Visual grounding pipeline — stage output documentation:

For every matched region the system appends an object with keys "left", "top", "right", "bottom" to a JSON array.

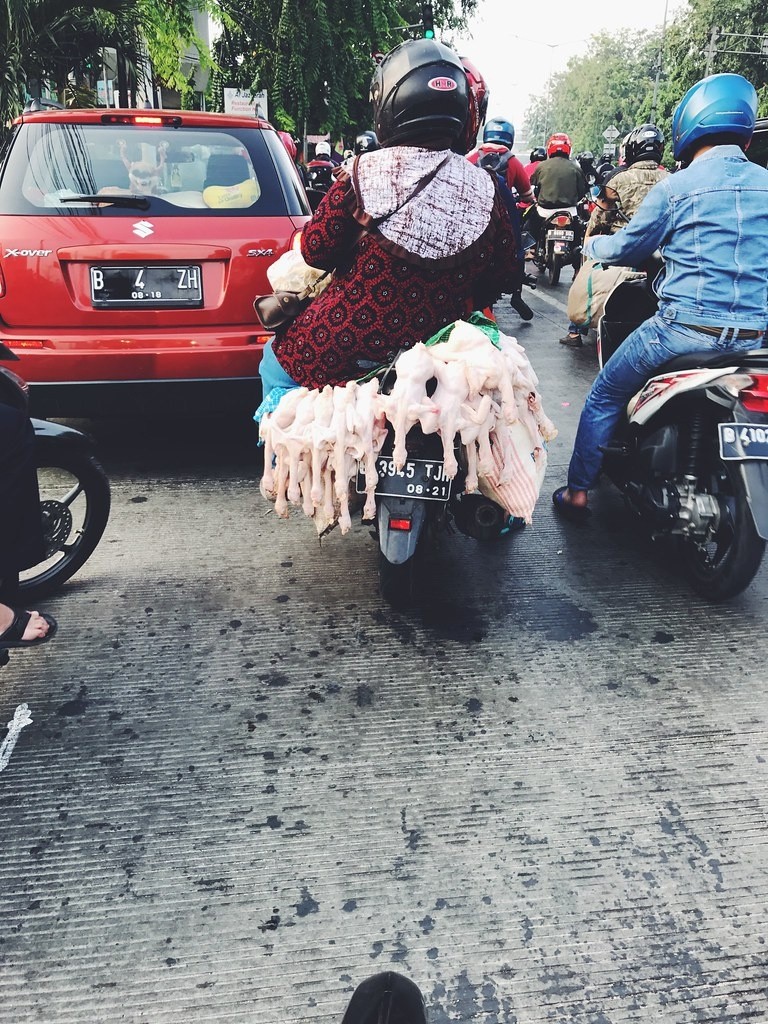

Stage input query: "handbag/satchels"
[{"left": 252, "top": 290, "right": 316, "bottom": 332}]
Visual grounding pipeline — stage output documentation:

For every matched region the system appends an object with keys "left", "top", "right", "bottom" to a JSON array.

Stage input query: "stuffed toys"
[
  {"left": 203, "top": 179, "right": 261, "bottom": 208},
  {"left": 120, "top": 146, "right": 164, "bottom": 194}
]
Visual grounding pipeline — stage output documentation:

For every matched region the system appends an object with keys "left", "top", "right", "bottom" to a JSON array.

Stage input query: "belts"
[{"left": 683, "top": 322, "right": 762, "bottom": 341}]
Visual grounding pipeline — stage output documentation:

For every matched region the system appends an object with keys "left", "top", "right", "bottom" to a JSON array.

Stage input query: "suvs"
[{"left": 0, "top": 102, "right": 327, "bottom": 420}]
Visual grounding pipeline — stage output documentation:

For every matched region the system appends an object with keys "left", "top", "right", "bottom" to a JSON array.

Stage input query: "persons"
[
  {"left": 259, "top": 39, "right": 673, "bottom": 418},
  {"left": 551, "top": 72, "right": 767, "bottom": 519},
  {"left": 0, "top": 403, "right": 58, "bottom": 650}
]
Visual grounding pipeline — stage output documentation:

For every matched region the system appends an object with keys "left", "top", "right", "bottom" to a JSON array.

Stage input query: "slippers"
[
  {"left": 553, "top": 486, "right": 591, "bottom": 519},
  {"left": 0, "top": 609, "right": 57, "bottom": 648}
]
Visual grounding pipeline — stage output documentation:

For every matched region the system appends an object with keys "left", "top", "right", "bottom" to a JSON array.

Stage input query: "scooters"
[
  {"left": 590, "top": 182, "right": 767, "bottom": 604},
  {"left": 490, "top": 187, "right": 599, "bottom": 312}
]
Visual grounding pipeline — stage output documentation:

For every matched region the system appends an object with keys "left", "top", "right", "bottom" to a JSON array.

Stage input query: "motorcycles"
[
  {"left": 351, "top": 410, "right": 510, "bottom": 610},
  {"left": 0, "top": 340, "right": 114, "bottom": 672}
]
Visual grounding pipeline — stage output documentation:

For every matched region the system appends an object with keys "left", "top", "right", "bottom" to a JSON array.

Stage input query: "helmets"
[
  {"left": 622, "top": 124, "right": 665, "bottom": 168},
  {"left": 530, "top": 147, "right": 547, "bottom": 164},
  {"left": 316, "top": 142, "right": 331, "bottom": 157},
  {"left": 369, "top": 39, "right": 469, "bottom": 151},
  {"left": 354, "top": 130, "right": 382, "bottom": 156},
  {"left": 575, "top": 151, "right": 593, "bottom": 170},
  {"left": 279, "top": 132, "right": 297, "bottom": 159},
  {"left": 602, "top": 154, "right": 611, "bottom": 164},
  {"left": 482, "top": 118, "right": 513, "bottom": 150},
  {"left": 672, "top": 73, "right": 757, "bottom": 161},
  {"left": 343, "top": 150, "right": 354, "bottom": 160},
  {"left": 547, "top": 132, "right": 570, "bottom": 160},
  {"left": 461, "top": 58, "right": 487, "bottom": 155}
]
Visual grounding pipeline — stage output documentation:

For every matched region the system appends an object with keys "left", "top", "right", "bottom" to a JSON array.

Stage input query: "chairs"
[
  {"left": 90, "top": 159, "right": 130, "bottom": 189},
  {"left": 206, "top": 154, "right": 250, "bottom": 187}
]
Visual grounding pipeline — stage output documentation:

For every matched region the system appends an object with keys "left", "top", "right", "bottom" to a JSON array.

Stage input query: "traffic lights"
[{"left": 422, "top": 4, "right": 435, "bottom": 39}]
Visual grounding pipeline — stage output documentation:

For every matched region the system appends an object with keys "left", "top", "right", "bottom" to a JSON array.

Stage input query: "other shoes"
[
  {"left": 511, "top": 296, "right": 534, "bottom": 320},
  {"left": 559, "top": 333, "right": 582, "bottom": 347}
]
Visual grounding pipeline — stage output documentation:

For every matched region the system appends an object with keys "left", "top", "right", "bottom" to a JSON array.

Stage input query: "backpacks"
[{"left": 475, "top": 149, "right": 515, "bottom": 187}]
